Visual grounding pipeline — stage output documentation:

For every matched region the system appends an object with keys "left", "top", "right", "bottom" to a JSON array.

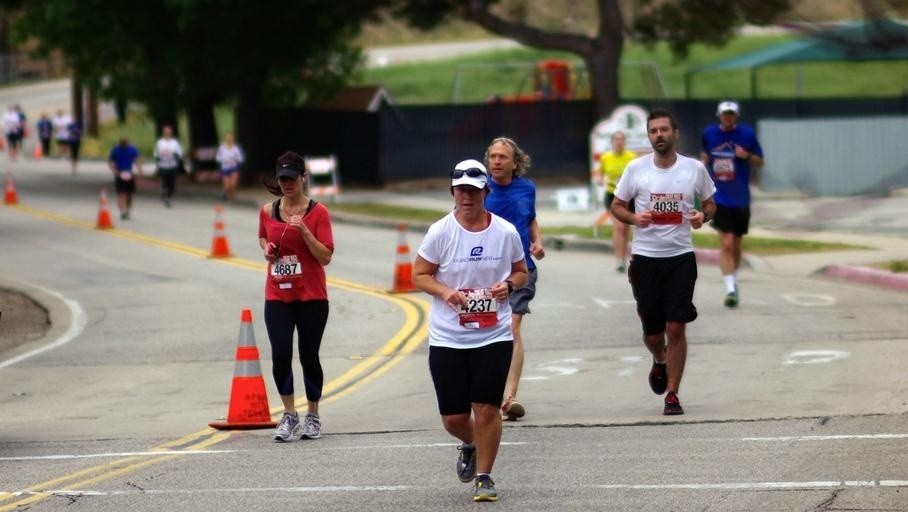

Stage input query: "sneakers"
[
  {"left": 471, "top": 473, "right": 499, "bottom": 503},
  {"left": 299, "top": 413, "right": 323, "bottom": 441},
  {"left": 272, "top": 411, "right": 301, "bottom": 443},
  {"left": 648, "top": 345, "right": 669, "bottom": 396},
  {"left": 663, "top": 391, "right": 684, "bottom": 415},
  {"left": 500, "top": 395, "right": 526, "bottom": 422},
  {"left": 723, "top": 284, "right": 740, "bottom": 307},
  {"left": 455, "top": 442, "right": 478, "bottom": 484}
]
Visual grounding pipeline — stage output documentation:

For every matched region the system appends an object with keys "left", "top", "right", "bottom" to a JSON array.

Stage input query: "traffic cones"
[
  {"left": 206, "top": 309, "right": 281, "bottom": 429},
  {"left": 94, "top": 184, "right": 117, "bottom": 230},
  {"left": 0, "top": 135, "right": 5, "bottom": 151},
  {"left": 23, "top": 122, "right": 29, "bottom": 139},
  {"left": 207, "top": 204, "right": 234, "bottom": 259},
  {"left": 384, "top": 223, "right": 425, "bottom": 294},
  {"left": 33, "top": 139, "right": 44, "bottom": 159},
  {"left": 3, "top": 170, "right": 22, "bottom": 205}
]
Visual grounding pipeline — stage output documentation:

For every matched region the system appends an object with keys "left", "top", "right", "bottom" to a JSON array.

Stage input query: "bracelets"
[
  {"left": 744, "top": 153, "right": 752, "bottom": 163},
  {"left": 505, "top": 279, "right": 514, "bottom": 295},
  {"left": 703, "top": 211, "right": 710, "bottom": 223}
]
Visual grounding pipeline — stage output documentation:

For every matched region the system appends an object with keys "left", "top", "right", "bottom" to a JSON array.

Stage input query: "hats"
[
  {"left": 451, "top": 158, "right": 488, "bottom": 189},
  {"left": 716, "top": 100, "right": 739, "bottom": 115},
  {"left": 275, "top": 151, "right": 306, "bottom": 181}
]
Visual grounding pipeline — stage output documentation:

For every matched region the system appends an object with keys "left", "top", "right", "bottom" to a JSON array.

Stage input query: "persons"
[
  {"left": 149, "top": 124, "right": 184, "bottom": 206},
  {"left": 107, "top": 136, "right": 142, "bottom": 220},
  {"left": 212, "top": 130, "right": 246, "bottom": 202},
  {"left": 1, "top": 101, "right": 84, "bottom": 178},
  {"left": 411, "top": 158, "right": 529, "bottom": 502},
  {"left": 594, "top": 132, "right": 637, "bottom": 273},
  {"left": 699, "top": 97, "right": 767, "bottom": 308},
  {"left": 258, "top": 151, "right": 335, "bottom": 442},
  {"left": 482, "top": 137, "right": 546, "bottom": 421},
  {"left": 609, "top": 111, "right": 718, "bottom": 414}
]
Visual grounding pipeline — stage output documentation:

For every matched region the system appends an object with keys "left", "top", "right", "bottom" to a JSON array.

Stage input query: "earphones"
[{"left": 302, "top": 177, "right": 304, "bottom": 182}]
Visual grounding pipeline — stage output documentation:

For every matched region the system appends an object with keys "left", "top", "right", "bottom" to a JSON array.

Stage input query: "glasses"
[{"left": 449, "top": 167, "right": 487, "bottom": 180}]
